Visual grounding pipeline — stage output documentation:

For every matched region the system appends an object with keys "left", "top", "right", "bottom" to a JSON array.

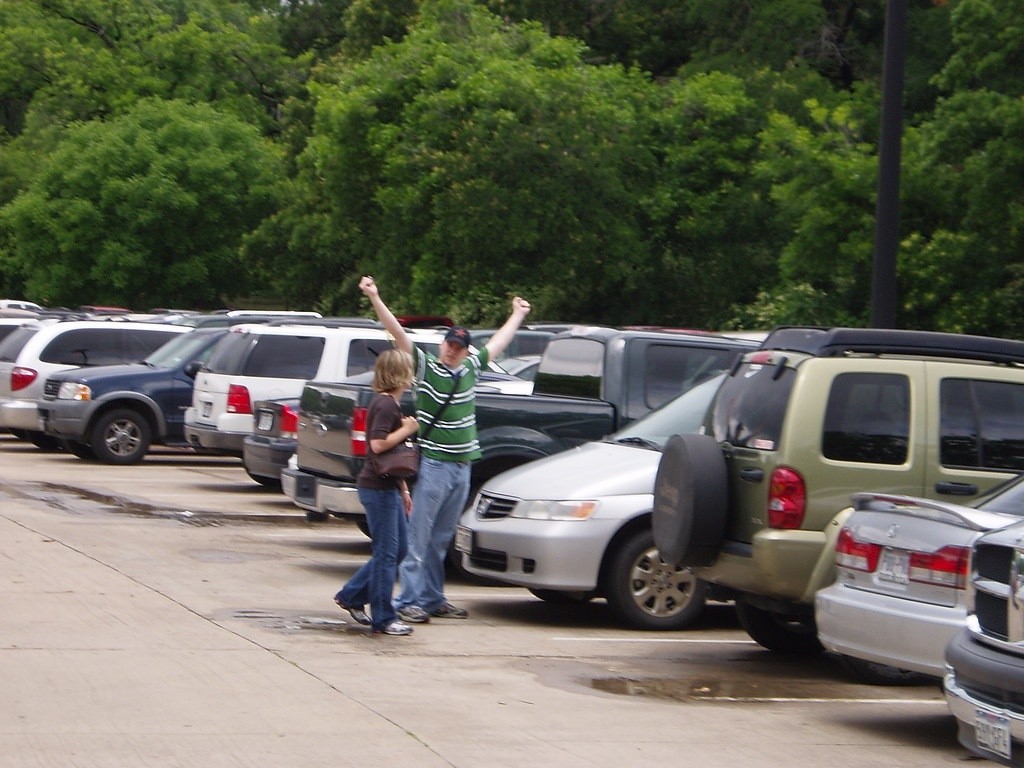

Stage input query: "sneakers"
[
  {"left": 334, "top": 594, "right": 372, "bottom": 625},
  {"left": 395, "top": 605, "right": 430, "bottom": 624},
  {"left": 372, "top": 619, "right": 413, "bottom": 636},
  {"left": 430, "top": 603, "right": 468, "bottom": 618}
]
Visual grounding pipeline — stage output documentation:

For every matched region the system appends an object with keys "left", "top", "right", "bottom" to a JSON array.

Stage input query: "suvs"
[
  {"left": 178, "top": 320, "right": 514, "bottom": 468},
  {"left": 652, "top": 328, "right": 1024, "bottom": 667}
]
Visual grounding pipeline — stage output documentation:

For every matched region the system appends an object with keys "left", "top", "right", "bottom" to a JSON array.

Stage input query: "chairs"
[{"left": 858, "top": 410, "right": 894, "bottom": 434}]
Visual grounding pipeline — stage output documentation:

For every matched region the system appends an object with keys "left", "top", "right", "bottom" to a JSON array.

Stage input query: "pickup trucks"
[{"left": 277, "top": 324, "right": 766, "bottom": 588}]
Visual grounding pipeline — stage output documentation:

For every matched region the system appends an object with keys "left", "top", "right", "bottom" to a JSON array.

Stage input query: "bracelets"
[{"left": 401, "top": 491, "right": 410, "bottom": 495}]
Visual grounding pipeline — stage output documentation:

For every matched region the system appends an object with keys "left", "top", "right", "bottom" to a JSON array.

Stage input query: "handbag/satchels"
[{"left": 371, "top": 397, "right": 419, "bottom": 479}]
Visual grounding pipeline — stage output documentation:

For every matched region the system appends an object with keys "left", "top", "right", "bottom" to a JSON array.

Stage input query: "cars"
[
  {"left": 461, "top": 326, "right": 555, "bottom": 382},
  {"left": 454, "top": 368, "right": 731, "bottom": 633},
  {"left": 812, "top": 470, "right": 1024, "bottom": 684},
  {"left": 243, "top": 372, "right": 369, "bottom": 485},
  {"left": 0, "top": 297, "right": 322, "bottom": 467},
  {"left": 941, "top": 517, "right": 1024, "bottom": 768}
]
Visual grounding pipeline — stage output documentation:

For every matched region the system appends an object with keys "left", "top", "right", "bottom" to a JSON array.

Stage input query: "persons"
[
  {"left": 359, "top": 276, "right": 531, "bottom": 623},
  {"left": 333, "top": 351, "right": 420, "bottom": 636}
]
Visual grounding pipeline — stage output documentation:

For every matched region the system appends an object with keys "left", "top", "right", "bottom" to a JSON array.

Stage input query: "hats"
[{"left": 445, "top": 326, "right": 471, "bottom": 347}]
